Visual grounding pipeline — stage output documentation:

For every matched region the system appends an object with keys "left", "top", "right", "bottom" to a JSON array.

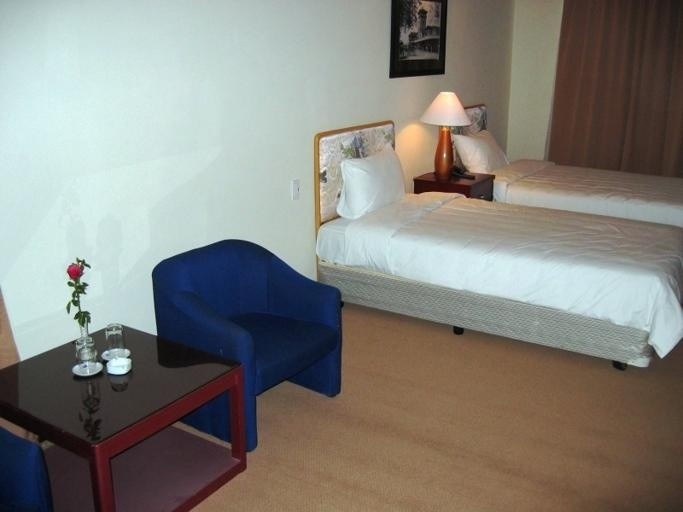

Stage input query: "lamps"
[{"left": 417, "top": 91, "right": 471, "bottom": 180}]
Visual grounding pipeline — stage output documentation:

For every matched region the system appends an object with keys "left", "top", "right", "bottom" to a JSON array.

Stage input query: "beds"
[
  {"left": 446, "top": 103, "right": 681, "bottom": 227},
  {"left": 312, "top": 120, "right": 682, "bottom": 371}
]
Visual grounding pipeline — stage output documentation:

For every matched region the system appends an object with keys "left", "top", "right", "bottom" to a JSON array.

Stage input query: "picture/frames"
[{"left": 388, "top": 0, "right": 448, "bottom": 78}]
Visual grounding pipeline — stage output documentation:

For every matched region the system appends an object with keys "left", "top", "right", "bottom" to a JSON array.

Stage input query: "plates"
[{"left": 72, "top": 348, "right": 131, "bottom": 378}]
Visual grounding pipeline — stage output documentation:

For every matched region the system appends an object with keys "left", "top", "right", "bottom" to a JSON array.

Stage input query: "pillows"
[
  {"left": 335, "top": 142, "right": 406, "bottom": 218},
  {"left": 453, "top": 128, "right": 510, "bottom": 174}
]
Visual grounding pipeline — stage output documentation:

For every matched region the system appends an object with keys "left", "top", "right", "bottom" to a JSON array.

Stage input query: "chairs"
[
  {"left": 0, "top": 424, "right": 55, "bottom": 511},
  {"left": 151, "top": 240, "right": 343, "bottom": 450}
]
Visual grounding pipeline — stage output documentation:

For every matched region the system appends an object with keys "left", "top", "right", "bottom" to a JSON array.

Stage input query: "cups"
[{"left": 74, "top": 324, "right": 126, "bottom": 374}]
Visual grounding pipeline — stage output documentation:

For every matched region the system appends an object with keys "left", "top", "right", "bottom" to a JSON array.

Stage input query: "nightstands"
[{"left": 414, "top": 171, "right": 496, "bottom": 202}]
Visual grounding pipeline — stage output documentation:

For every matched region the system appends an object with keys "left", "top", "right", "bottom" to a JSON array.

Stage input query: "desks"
[{"left": 0, "top": 322, "right": 246, "bottom": 512}]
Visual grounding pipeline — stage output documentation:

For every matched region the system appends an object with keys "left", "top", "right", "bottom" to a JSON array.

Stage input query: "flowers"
[{"left": 65, "top": 257, "right": 93, "bottom": 325}]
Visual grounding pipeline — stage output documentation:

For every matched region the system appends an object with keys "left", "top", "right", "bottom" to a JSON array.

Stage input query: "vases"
[{"left": 74, "top": 319, "right": 98, "bottom": 372}]
